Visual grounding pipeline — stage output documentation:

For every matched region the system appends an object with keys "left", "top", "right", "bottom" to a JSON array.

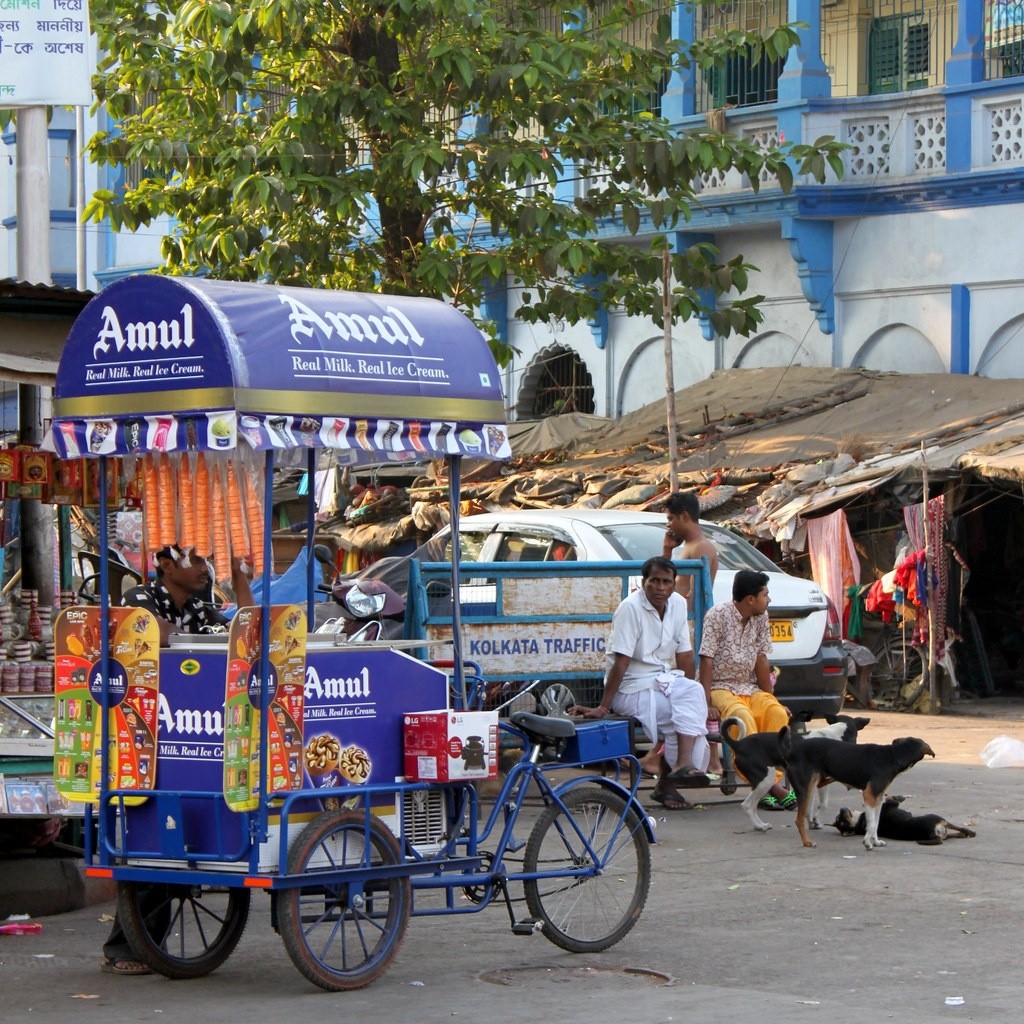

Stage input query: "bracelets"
[{"left": 598, "top": 705, "right": 610, "bottom": 716}]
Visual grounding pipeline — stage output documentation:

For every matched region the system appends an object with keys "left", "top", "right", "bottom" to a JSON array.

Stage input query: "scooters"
[{"left": 79, "top": 539, "right": 451, "bottom": 641}]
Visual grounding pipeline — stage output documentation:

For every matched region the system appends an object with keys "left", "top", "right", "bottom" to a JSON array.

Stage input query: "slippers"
[
  {"left": 648, "top": 788, "right": 695, "bottom": 810},
  {"left": 667, "top": 764, "right": 711, "bottom": 787},
  {"left": 780, "top": 789, "right": 798, "bottom": 810},
  {"left": 757, "top": 796, "right": 785, "bottom": 811},
  {"left": 101, "top": 956, "right": 152, "bottom": 974},
  {"left": 620, "top": 757, "right": 662, "bottom": 779}
]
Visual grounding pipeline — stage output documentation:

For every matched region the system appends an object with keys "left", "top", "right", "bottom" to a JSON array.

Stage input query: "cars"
[{"left": 413, "top": 509, "right": 849, "bottom": 717}]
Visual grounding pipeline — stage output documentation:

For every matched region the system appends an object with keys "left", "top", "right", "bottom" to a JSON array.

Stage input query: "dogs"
[{"left": 719, "top": 715, "right": 977, "bottom": 851}]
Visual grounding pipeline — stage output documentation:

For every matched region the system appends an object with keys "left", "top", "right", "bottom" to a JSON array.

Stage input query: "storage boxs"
[
  {"left": 564, "top": 720, "right": 631, "bottom": 765},
  {"left": 402, "top": 708, "right": 499, "bottom": 784}
]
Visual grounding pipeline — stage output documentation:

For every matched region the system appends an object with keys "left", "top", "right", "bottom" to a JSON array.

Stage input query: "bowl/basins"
[{"left": 0, "top": 587, "right": 79, "bottom": 694}]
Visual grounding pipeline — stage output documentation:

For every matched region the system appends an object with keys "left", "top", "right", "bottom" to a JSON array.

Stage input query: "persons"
[
  {"left": 696, "top": 568, "right": 796, "bottom": 810},
  {"left": 661, "top": 492, "right": 718, "bottom": 645},
  {"left": 565, "top": 557, "right": 712, "bottom": 808},
  {"left": 99, "top": 540, "right": 257, "bottom": 975}
]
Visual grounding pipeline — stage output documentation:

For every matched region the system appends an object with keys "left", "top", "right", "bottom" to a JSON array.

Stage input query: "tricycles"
[{"left": 40, "top": 273, "right": 658, "bottom": 992}]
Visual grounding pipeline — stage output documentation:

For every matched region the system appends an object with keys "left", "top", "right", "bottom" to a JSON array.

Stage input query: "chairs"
[{"left": 79, "top": 549, "right": 142, "bottom": 608}]
